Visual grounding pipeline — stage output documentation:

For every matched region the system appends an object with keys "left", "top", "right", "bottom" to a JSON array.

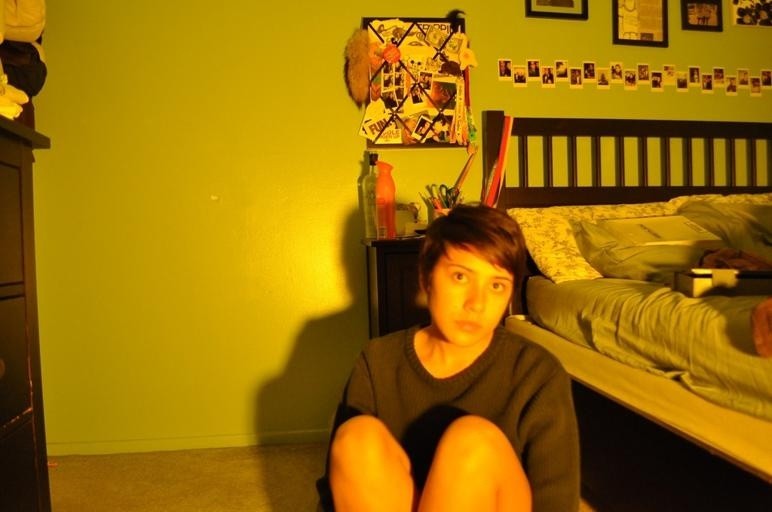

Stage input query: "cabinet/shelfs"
[{"left": 0, "top": 116, "right": 52, "bottom": 509}]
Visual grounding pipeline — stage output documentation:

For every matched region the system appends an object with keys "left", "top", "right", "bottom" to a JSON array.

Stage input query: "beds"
[{"left": 480, "top": 108, "right": 771, "bottom": 512}]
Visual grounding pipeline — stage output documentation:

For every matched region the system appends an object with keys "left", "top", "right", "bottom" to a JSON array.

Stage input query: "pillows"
[{"left": 505, "top": 191, "right": 771, "bottom": 287}]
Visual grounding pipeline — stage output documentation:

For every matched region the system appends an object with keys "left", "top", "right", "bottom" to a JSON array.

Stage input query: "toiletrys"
[
  {"left": 361, "top": 151, "right": 378, "bottom": 237},
  {"left": 373, "top": 160, "right": 397, "bottom": 238}
]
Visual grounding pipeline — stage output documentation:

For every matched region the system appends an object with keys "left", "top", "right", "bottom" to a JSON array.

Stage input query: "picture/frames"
[{"left": 523, "top": 0, "right": 723, "bottom": 48}]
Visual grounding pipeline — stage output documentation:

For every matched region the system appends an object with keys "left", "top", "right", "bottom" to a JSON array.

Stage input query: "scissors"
[{"left": 440, "top": 184, "right": 459, "bottom": 208}]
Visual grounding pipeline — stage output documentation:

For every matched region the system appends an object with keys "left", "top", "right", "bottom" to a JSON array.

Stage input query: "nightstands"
[{"left": 360, "top": 235, "right": 431, "bottom": 340}]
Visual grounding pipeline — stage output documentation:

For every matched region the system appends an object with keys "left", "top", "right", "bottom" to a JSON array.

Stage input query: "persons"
[
  {"left": 696, "top": 5, "right": 712, "bottom": 25},
  {"left": 314, "top": 202, "right": 582, "bottom": 512},
  {"left": 375, "top": 25, "right": 456, "bottom": 143},
  {"left": 499, "top": 61, "right": 771, "bottom": 93}
]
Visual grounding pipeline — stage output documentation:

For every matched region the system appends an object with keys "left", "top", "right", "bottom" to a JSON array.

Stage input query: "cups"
[{"left": 433, "top": 208, "right": 451, "bottom": 221}]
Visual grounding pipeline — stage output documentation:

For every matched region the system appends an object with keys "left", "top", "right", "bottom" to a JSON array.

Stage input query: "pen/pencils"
[{"left": 429, "top": 184, "right": 452, "bottom": 208}]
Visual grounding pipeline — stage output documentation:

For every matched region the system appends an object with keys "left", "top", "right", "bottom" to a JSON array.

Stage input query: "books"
[
  {"left": 673, "top": 268, "right": 772, "bottom": 297},
  {"left": 596, "top": 214, "right": 725, "bottom": 248}
]
